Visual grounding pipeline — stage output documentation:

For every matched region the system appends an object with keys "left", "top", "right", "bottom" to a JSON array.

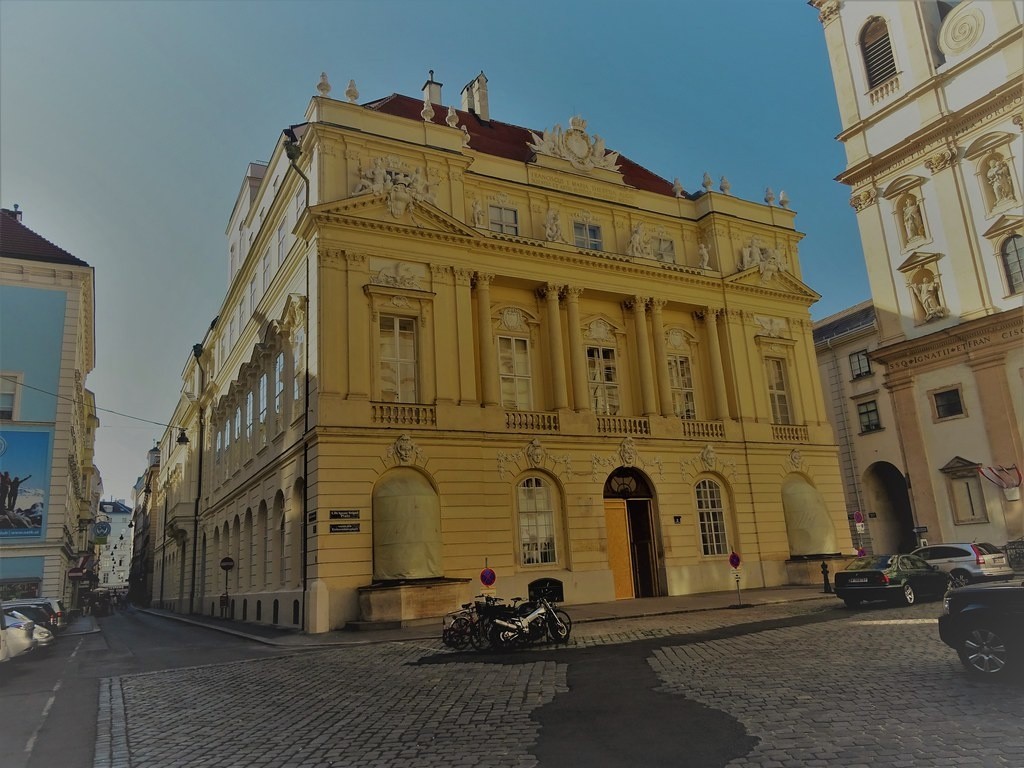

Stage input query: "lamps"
[
  {"left": 176, "top": 432, "right": 190, "bottom": 445},
  {"left": 127, "top": 521, "right": 134, "bottom": 528},
  {"left": 143, "top": 484, "right": 152, "bottom": 494}
]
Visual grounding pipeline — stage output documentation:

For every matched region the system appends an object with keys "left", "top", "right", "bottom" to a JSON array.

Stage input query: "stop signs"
[{"left": 220, "top": 557, "right": 234, "bottom": 571}]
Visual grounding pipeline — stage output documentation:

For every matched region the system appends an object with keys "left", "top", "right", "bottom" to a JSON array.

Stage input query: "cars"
[
  {"left": 0, "top": 596, "right": 72, "bottom": 663},
  {"left": 833, "top": 553, "right": 950, "bottom": 611},
  {"left": 937, "top": 583, "right": 1024, "bottom": 677}
]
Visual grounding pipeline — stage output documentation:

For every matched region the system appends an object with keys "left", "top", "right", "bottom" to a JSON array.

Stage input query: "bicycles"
[{"left": 440, "top": 582, "right": 572, "bottom": 655}]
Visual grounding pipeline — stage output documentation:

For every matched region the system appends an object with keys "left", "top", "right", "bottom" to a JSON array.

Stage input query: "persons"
[
  {"left": 698, "top": 243, "right": 713, "bottom": 270},
  {"left": 471, "top": 200, "right": 483, "bottom": 228},
  {"left": 408, "top": 167, "right": 438, "bottom": 207},
  {"left": 544, "top": 209, "right": 562, "bottom": 242},
  {"left": 901, "top": 196, "right": 925, "bottom": 241},
  {"left": 911, "top": 277, "right": 944, "bottom": 324},
  {"left": 351, "top": 156, "right": 393, "bottom": 198},
  {"left": 630, "top": 228, "right": 645, "bottom": 258},
  {"left": 987, "top": 159, "right": 1014, "bottom": 205},
  {"left": 82, "top": 588, "right": 128, "bottom": 618},
  {"left": 0, "top": 471, "right": 32, "bottom": 511},
  {"left": 749, "top": 234, "right": 765, "bottom": 266}
]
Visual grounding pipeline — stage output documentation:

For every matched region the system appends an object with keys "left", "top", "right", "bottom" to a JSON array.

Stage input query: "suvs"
[{"left": 907, "top": 537, "right": 1017, "bottom": 584}]
